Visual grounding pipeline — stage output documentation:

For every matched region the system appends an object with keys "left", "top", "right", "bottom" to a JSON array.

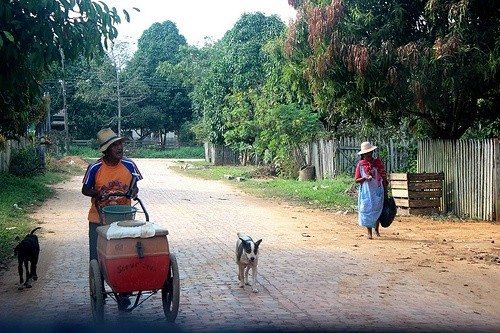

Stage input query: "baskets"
[{"left": 102, "top": 205, "right": 137, "bottom": 225}]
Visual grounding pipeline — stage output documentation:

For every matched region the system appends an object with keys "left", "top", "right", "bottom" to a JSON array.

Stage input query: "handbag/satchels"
[{"left": 379, "top": 197, "right": 397, "bottom": 227}]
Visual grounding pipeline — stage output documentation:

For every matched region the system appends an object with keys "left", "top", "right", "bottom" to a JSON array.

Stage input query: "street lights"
[{"left": 58, "top": 79, "right": 69, "bottom": 156}]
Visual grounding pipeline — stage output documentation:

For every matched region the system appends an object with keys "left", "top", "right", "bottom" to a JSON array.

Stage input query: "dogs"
[
  {"left": 235, "top": 232, "right": 263, "bottom": 293},
  {"left": 14, "top": 227, "right": 42, "bottom": 290}
]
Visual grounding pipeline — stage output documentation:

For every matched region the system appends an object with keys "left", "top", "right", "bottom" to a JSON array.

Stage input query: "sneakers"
[{"left": 117, "top": 297, "right": 131, "bottom": 310}]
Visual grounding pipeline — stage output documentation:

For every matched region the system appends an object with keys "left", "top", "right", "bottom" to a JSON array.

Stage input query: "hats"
[
  {"left": 358, "top": 142, "right": 378, "bottom": 155},
  {"left": 96, "top": 127, "right": 129, "bottom": 154}
]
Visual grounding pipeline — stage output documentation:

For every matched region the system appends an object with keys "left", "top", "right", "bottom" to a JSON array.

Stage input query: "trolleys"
[{"left": 88, "top": 192, "right": 180, "bottom": 323}]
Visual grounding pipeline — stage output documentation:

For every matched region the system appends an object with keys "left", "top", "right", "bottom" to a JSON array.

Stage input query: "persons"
[
  {"left": 81, "top": 127, "right": 143, "bottom": 311},
  {"left": 354, "top": 142, "right": 389, "bottom": 239}
]
